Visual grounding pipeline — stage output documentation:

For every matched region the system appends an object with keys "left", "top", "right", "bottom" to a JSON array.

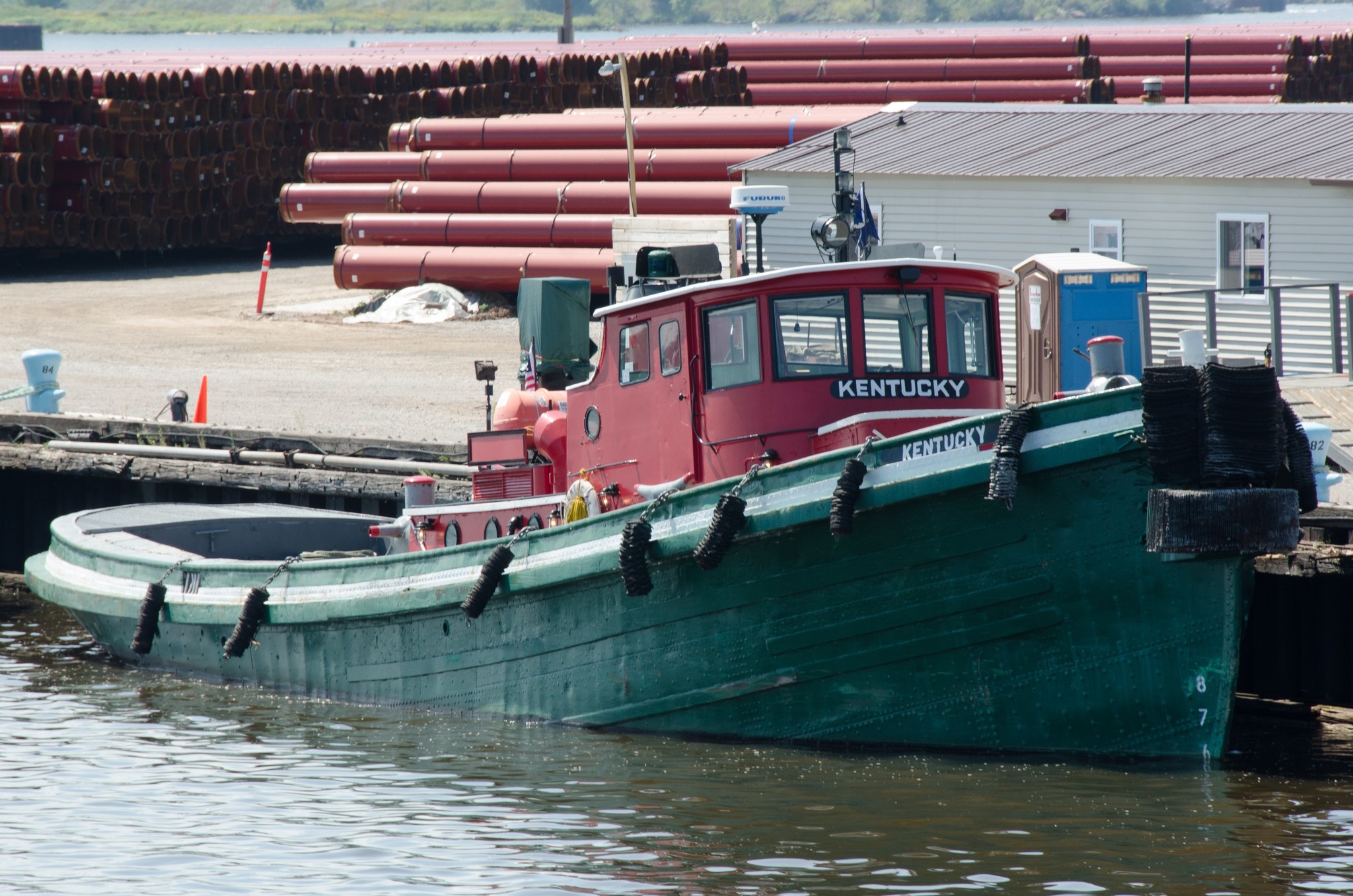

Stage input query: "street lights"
[
  {"left": 597, "top": 60, "right": 640, "bottom": 219},
  {"left": 832, "top": 127, "right": 853, "bottom": 263}
]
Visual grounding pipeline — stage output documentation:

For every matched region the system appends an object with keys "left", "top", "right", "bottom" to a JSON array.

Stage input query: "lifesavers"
[{"left": 561, "top": 478, "right": 610, "bottom": 524}]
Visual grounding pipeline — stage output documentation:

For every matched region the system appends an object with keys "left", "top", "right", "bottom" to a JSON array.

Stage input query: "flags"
[{"left": 525, "top": 342, "right": 539, "bottom": 391}]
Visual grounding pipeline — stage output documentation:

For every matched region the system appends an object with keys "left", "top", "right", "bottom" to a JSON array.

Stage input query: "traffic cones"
[{"left": 194, "top": 376, "right": 208, "bottom": 424}]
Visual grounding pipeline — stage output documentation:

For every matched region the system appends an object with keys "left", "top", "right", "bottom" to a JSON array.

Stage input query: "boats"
[{"left": 26, "top": 183, "right": 1257, "bottom": 766}]
